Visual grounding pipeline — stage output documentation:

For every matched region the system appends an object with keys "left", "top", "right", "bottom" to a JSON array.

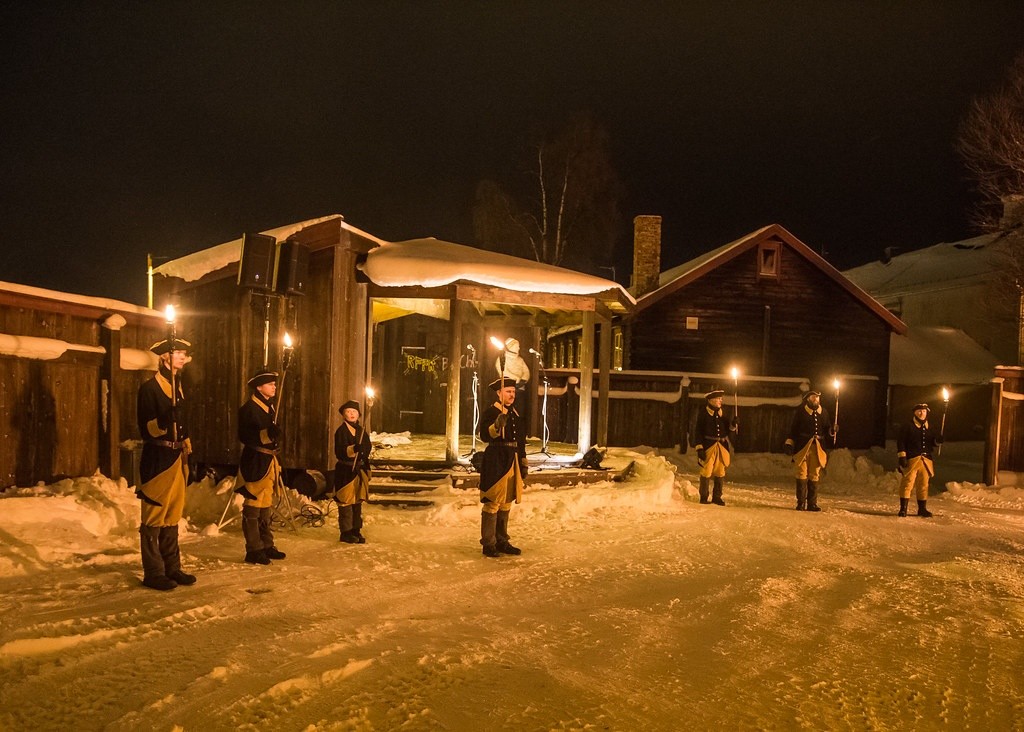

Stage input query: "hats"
[
  {"left": 339, "top": 400, "right": 362, "bottom": 417},
  {"left": 912, "top": 401, "right": 931, "bottom": 414},
  {"left": 248, "top": 371, "right": 279, "bottom": 387},
  {"left": 149, "top": 334, "right": 192, "bottom": 355},
  {"left": 802, "top": 389, "right": 821, "bottom": 402},
  {"left": 488, "top": 376, "right": 517, "bottom": 391},
  {"left": 703, "top": 389, "right": 725, "bottom": 400}
]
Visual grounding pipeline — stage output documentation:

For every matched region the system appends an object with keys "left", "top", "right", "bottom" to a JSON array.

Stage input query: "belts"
[
  {"left": 797, "top": 432, "right": 826, "bottom": 440},
  {"left": 338, "top": 458, "right": 365, "bottom": 466},
  {"left": 910, "top": 451, "right": 931, "bottom": 456},
  {"left": 489, "top": 440, "right": 518, "bottom": 448},
  {"left": 246, "top": 443, "right": 281, "bottom": 456},
  {"left": 143, "top": 437, "right": 183, "bottom": 450},
  {"left": 704, "top": 435, "right": 729, "bottom": 441}
]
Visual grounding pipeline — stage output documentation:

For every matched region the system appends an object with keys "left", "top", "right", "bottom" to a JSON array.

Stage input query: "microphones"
[
  {"left": 529, "top": 348, "right": 541, "bottom": 357},
  {"left": 467, "top": 344, "right": 475, "bottom": 352}
]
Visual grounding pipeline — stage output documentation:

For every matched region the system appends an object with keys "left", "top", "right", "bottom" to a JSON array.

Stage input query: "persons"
[
  {"left": 137, "top": 336, "right": 197, "bottom": 589},
  {"left": 786, "top": 388, "right": 839, "bottom": 512},
  {"left": 479, "top": 377, "right": 528, "bottom": 557},
  {"left": 334, "top": 400, "right": 373, "bottom": 543},
  {"left": 694, "top": 389, "right": 740, "bottom": 507},
  {"left": 896, "top": 402, "right": 944, "bottom": 517},
  {"left": 236, "top": 371, "right": 286, "bottom": 565},
  {"left": 496, "top": 338, "right": 530, "bottom": 446}
]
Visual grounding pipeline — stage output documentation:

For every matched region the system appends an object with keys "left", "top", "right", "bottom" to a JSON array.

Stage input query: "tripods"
[
  {"left": 462, "top": 352, "right": 479, "bottom": 460},
  {"left": 525, "top": 356, "right": 556, "bottom": 459}
]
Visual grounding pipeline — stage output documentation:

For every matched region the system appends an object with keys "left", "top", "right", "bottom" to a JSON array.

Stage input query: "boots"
[{"left": 139, "top": 475, "right": 937, "bottom": 591}]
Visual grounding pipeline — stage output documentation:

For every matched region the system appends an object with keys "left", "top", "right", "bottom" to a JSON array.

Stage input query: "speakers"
[{"left": 237, "top": 232, "right": 309, "bottom": 296}]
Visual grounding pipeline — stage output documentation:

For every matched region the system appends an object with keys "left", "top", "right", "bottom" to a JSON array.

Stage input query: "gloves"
[
  {"left": 267, "top": 424, "right": 281, "bottom": 440},
  {"left": 830, "top": 425, "right": 839, "bottom": 435},
  {"left": 697, "top": 448, "right": 706, "bottom": 461},
  {"left": 784, "top": 444, "right": 794, "bottom": 456},
  {"left": 731, "top": 416, "right": 741, "bottom": 428},
  {"left": 898, "top": 457, "right": 908, "bottom": 468},
  {"left": 520, "top": 465, "right": 528, "bottom": 479},
  {"left": 935, "top": 434, "right": 944, "bottom": 445},
  {"left": 354, "top": 444, "right": 364, "bottom": 453},
  {"left": 157, "top": 406, "right": 182, "bottom": 430},
  {"left": 495, "top": 413, "right": 510, "bottom": 428}
]
